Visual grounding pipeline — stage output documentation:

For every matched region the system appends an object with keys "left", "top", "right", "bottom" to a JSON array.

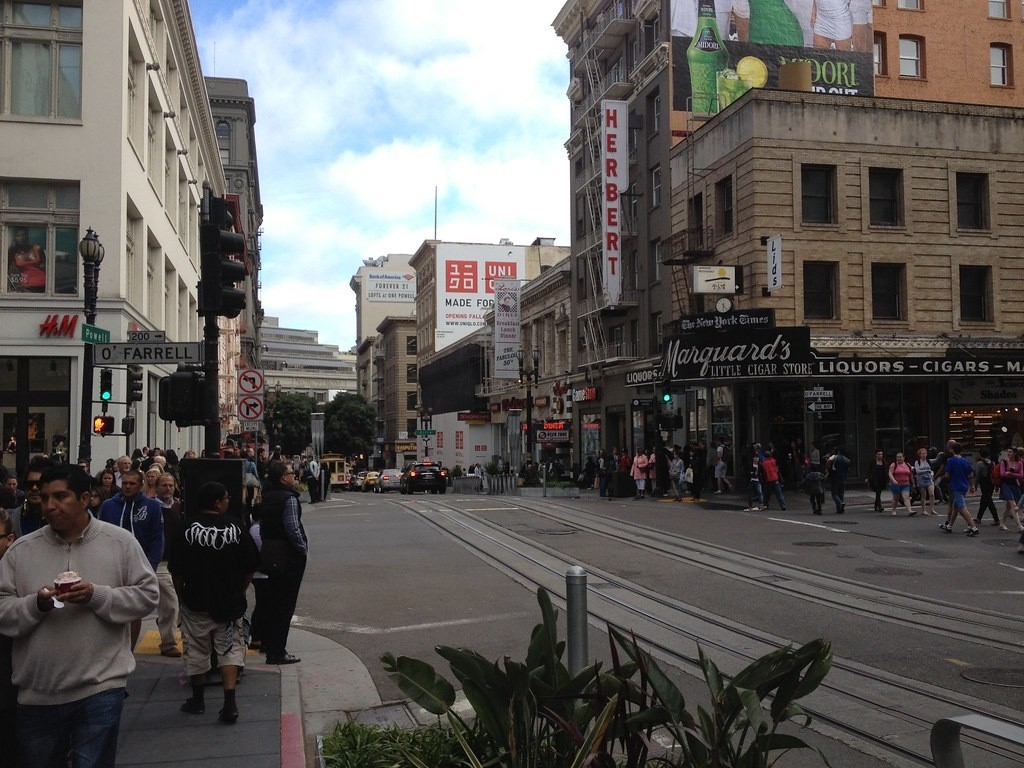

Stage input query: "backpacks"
[
  {"left": 978, "top": 459, "right": 995, "bottom": 480},
  {"left": 992, "top": 459, "right": 1007, "bottom": 485},
  {"left": 721, "top": 445, "right": 730, "bottom": 462}
]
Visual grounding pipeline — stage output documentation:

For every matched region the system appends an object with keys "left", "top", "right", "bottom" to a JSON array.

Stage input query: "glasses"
[
  {"left": 221, "top": 496, "right": 231, "bottom": 501},
  {"left": 286, "top": 471, "right": 295, "bottom": 476}
]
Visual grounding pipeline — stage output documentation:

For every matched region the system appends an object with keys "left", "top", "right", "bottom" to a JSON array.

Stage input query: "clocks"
[{"left": 715, "top": 298, "right": 733, "bottom": 312}]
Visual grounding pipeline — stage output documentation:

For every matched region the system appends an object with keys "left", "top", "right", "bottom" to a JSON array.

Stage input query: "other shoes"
[
  {"left": 749, "top": 501, "right": 938, "bottom": 517},
  {"left": 973, "top": 519, "right": 981, "bottom": 523},
  {"left": 181, "top": 698, "right": 204, "bottom": 714},
  {"left": 991, "top": 522, "right": 1000, "bottom": 526},
  {"left": 1018, "top": 543, "right": 1024, "bottom": 551},
  {"left": 219, "top": 707, "right": 239, "bottom": 721},
  {"left": 161, "top": 648, "right": 181, "bottom": 657},
  {"left": 714, "top": 490, "right": 722, "bottom": 493},
  {"left": 266, "top": 652, "right": 301, "bottom": 663},
  {"left": 249, "top": 641, "right": 261, "bottom": 649},
  {"left": 1000, "top": 525, "right": 1010, "bottom": 531},
  {"left": 1018, "top": 526, "right": 1024, "bottom": 532}
]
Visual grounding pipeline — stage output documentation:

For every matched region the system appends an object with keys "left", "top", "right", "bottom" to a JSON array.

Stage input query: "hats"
[
  {"left": 753, "top": 443, "right": 761, "bottom": 449},
  {"left": 765, "top": 451, "right": 771, "bottom": 455}
]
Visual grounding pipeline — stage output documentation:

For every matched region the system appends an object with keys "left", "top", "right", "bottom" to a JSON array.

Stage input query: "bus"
[{"left": 318, "top": 453, "right": 348, "bottom": 491}]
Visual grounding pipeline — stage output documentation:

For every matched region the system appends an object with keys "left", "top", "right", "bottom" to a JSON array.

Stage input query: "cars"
[
  {"left": 373, "top": 469, "right": 404, "bottom": 493},
  {"left": 346, "top": 465, "right": 380, "bottom": 493}
]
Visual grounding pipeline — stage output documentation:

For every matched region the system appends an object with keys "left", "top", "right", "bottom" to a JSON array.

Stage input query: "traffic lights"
[
  {"left": 201, "top": 223, "right": 247, "bottom": 309},
  {"left": 100, "top": 369, "right": 113, "bottom": 400},
  {"left": 660, "top": 375, "right": 673, "bottom": 403},
  {"left": 127, "top": 365, "right": 144, "bottom": 402},
  {"left": 94, "top": 415, "right": 109, "bottom": 432},
  {"left": 210, "top": 197, "right": 241, "bottom": 319}
]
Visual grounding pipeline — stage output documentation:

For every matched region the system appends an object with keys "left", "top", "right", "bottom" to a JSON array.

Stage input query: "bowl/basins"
[{"left": 53, "top": 577, "right": 82, "bottom": 596}]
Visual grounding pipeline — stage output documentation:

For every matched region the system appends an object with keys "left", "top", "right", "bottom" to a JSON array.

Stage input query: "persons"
[
  {"left": 939, "top": 437, "right": 1024, "bottom": 554},
  {"left": 7, "top": 419, "right": 38, "bottom": 450},
  {"left": 1, "top": 448, "right": 333, "bottom": 648},
  {"left": 670, "top": 0, "right": 873, "bottom": 53},
  {"left": 744, "top": 441, "right": 786, "bottom": 510},
  {"left": 55, "top": 441, "right": 68, "bottom": 463},
  {"left": 165, "top": 481, "right": 261, "bottom": 723},
  {"left": 9, "top": 227, "right": 69, "bottom": 293},
  {"left": 1, "top": 465, "right": 161, "bottom": 768},
  {"left": 865, "top": 447, "right": 943, "bottom": 515},
  {"left": 597, "top": 447, "right": 656, "bottom": 497},
  {"left": 259, "top": 464, "right": 308, "bottom": 666},
  {"left": 790, "top": 437, "right": 851, "bottom": 515},
  {"left": 463, "top": 463, "right": 484, "bottom": 489},
  {"left": 663, "top": 437, "right": 732, "bottom": 500}
]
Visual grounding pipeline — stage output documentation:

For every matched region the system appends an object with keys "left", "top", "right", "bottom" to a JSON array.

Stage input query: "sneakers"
[
  {"left": 967, "top": 529, "right": 979, "bottom": 537},
  {"left": 939, "top": 523, "right": 952, "bottom": 533},
  {"left": 963, "top": 527, "right": 971, "bottom": 533}
]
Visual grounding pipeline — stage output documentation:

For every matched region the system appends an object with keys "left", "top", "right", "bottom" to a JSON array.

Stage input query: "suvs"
[{"left": 399, "top": 461, "right": 447, "bottom": 495}]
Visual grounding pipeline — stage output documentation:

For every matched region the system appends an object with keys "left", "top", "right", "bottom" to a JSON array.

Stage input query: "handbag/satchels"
[
  {"left": 639, "top": 467, "right": 646, "bottom": 473},
  {"left": 685, "top": 468, "right": 693, "bottom": 483},
  {"left": 680, "top": 471, "right": 686, "bottom": 482},
  {"left": 671, "top": 473, "right": 679, "bottom": 481}
]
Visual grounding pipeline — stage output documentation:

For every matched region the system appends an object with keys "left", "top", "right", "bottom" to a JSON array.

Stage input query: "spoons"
[{"left": 43, "top": 587, "right": 64, "bottom": 609}]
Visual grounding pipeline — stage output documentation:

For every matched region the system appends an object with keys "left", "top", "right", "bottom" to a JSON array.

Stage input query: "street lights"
[
  {"left": 515, "top": 344, "right": 542, "bottom": 465},
  {"left": 78, "top": 227, "right": 107, "bottom": 474},
  {"left": 418, "top": 405, "right": 433, "bottom": 456}
]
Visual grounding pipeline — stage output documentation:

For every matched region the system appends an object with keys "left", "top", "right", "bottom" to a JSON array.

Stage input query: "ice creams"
[{"left": 53, "top": 571, "right": 82, "bottom": 583}]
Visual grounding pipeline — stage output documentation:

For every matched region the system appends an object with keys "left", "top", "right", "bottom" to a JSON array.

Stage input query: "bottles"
[{"left": 1010, "top": 464, "right": 1016, "bottom": 473}]
